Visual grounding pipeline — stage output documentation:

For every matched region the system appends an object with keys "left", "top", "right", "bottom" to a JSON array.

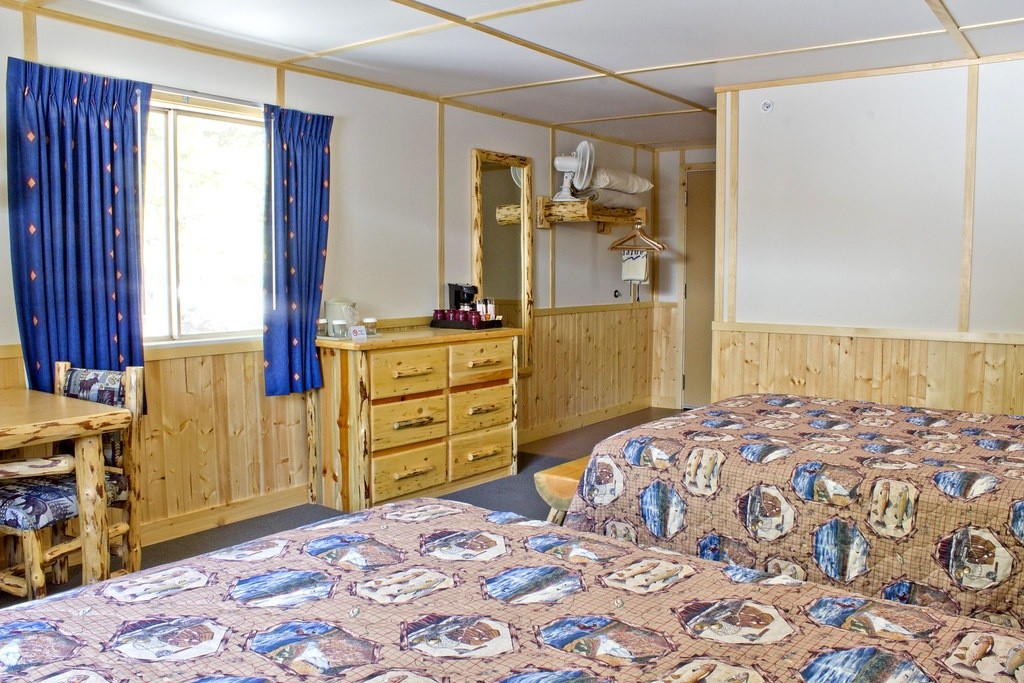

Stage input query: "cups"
[
  {"left": 433, "top": 309, "right": 445, "bottom": 320},
  {"left": 445, "top": 309, "right": 457, "bottom": 321},
  {"left": 331, "top": 320, "right": 348, "bottom": 337},
  {"left": 316, "top": 318, "right": 328, "bottom": 336},
  {"left": 468, "top": 311, "right": 481, "bottom": 327},
  {"left": 363, "top": 318, "right": 377, "bottom": 335},
  {"left": 455, "top": 310, "right": 468, "bottom": 321}
]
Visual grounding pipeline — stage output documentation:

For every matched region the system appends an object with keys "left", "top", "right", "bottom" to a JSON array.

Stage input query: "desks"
[{"left": 0, "top": 389, "right": 134, "bottom": 587}]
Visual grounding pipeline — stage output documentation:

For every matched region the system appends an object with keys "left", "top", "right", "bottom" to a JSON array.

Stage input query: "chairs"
[{"left": 0, "top": 360, "right": 147, "bottom": 605}]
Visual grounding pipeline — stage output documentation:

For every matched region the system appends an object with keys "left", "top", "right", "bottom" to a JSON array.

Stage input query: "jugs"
[
  {"left": 475, "top": 296, "right": 496, "bottom": 320},
  {"left": 324, "top": 295, "right": 356, "bottom": 337}
]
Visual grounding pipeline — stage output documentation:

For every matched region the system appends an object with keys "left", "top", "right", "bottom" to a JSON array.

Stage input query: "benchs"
[{"left": 532, "top": 451, "right": 590, "bottom": 526}]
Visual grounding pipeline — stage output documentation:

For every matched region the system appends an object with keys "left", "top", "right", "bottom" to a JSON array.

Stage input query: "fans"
[{"left": 552, "top": 140, "right": 595, "bottom": 201}]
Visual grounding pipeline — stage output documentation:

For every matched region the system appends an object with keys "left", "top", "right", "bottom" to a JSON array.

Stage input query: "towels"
[{"left": 620, "top": 250, "right": 650, "bottom": 285}]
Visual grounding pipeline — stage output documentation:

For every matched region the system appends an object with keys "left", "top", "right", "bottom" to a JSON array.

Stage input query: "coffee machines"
[{"left": 448, "top": 282, "right": 478, "bottom": 311}]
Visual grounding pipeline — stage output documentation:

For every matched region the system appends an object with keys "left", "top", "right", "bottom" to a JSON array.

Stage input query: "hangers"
[{"left": 606, "top": 218, "right": 667, "bottom": 254}]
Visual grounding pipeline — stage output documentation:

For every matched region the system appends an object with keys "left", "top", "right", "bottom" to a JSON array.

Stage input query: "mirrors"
[{"left": 470, "top": 148, "right": 537, "bottom": 379}]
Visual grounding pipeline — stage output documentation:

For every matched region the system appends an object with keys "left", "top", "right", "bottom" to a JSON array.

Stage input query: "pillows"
[{"left": 588, "top": 166, "right": 654, "bottom": 193}]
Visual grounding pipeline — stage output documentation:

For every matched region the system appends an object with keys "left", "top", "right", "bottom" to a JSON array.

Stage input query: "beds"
[
  {"left": 0, "top": 498, "right": 1023, "bottom": 683},
  {"left": 557, "top": 391, "right": 1024, "bottom": 632}
]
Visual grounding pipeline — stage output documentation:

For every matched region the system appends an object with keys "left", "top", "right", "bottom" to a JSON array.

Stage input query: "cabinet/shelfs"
[{"left": 305, "top": 323, "right": 525, "bottom": 516}]
